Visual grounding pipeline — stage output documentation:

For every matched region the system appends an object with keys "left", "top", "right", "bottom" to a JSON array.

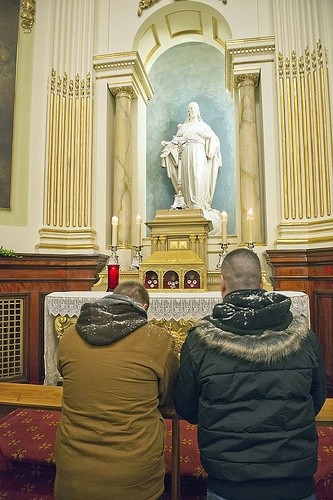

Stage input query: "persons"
[
  {"left": 159, "top": 100, "right": 222, "bottom": 211},
  {"left": 172, "top": 248, "right": 326, "bottom": 500},
  {"left": 52, "top": 281, "right": 179, "bottom": 500}
]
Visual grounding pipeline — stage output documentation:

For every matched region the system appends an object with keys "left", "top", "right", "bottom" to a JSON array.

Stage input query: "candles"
[
  {"left": 247, "top": 208, "right": 254, "bottom": 242},
  {"left": 135, "top": 214, "right": 141, "bottom": 244},
  {"left": 220, "top": 210, "right": 229, "bottom": 242},
  {"left": 111, "top": 216, "right": 119, "bottom": 246}
]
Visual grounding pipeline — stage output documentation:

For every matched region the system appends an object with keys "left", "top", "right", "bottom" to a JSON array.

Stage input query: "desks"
[{"left": 43, "top": 291, "right": 311, "bottom": 386}]
